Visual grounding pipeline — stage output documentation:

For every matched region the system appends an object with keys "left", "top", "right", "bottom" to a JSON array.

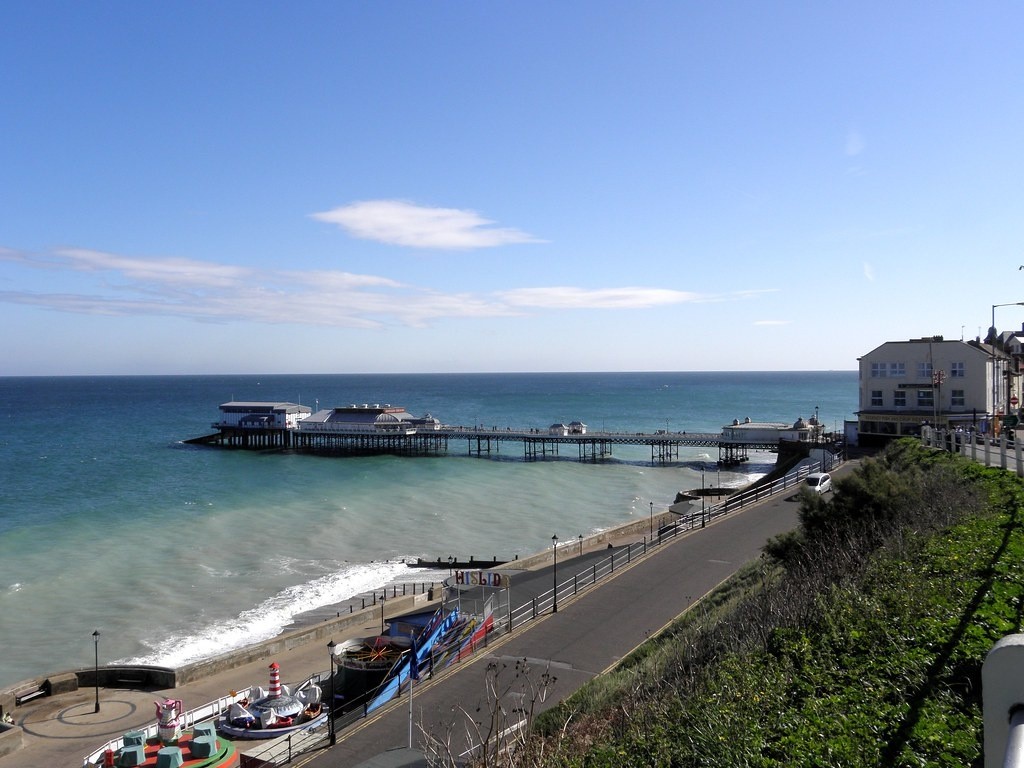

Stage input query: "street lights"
[
  {"left": 92, "top": 629, "right": 101, "bottom": 717},
  {"left": 579, "top": 534, "right": 583, "bottom": 557},
  {"left": 698, "top": 467, "right": 705, "bottom": 528},
  {"left": 821, "top": 423, "right": 827, "bottom": 473},
  {"left": 551, "top": 534, "right": 559, "bottom": 613},
  {"left": 718, "top": 468, "right": 720, "bottom": 501},
  {"left": 650, "top": 502, "right": 653, "bottom": 540}
]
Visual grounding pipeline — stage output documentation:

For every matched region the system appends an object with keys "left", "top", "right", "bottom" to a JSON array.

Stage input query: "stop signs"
[{"left": 1010, "top": 397, "right": 1018, "bottom": 404}]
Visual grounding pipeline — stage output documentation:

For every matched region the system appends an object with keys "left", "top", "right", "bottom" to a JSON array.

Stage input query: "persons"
[
  {"left": 683, "top": 430, "right": 686, "bottom": 434},
  {"left": 821, "top": 429, "right": 840, "bottom": 443},
  {"left": 678, "top": 431, "right": 681, "bottom": 434},
  {"left": 531, "top": 428, "right": 533, "bottom": 432},
  {"left": 536, "top": 428, "right": 539, "bottom": 434}
]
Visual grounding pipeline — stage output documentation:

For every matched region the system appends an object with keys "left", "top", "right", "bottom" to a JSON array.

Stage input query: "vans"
[{"left": 800, "top": 471, "right": 833, "bottom": 496}]
